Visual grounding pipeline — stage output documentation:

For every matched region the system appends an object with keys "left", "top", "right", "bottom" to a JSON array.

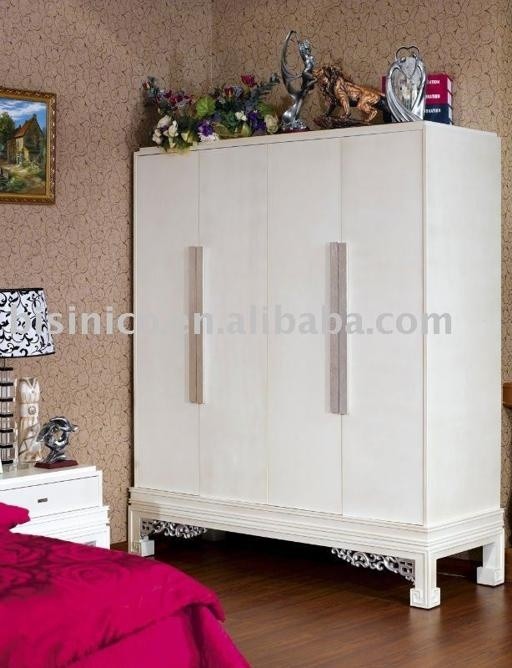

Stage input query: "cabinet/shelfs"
[{"left": 131, "top": 120, "right": 505, "bottom": 610}]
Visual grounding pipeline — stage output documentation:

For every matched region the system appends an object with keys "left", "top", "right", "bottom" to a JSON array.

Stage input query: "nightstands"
[{"left": 0, "top": 459, "right": 110, "bottom": 549}]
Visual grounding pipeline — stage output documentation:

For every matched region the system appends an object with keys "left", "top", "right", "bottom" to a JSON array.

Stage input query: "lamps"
[{"left": 1, "top": 288, "right": 56, "bottom": 466}]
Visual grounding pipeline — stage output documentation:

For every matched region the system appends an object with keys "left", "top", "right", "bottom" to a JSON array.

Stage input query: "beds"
[{"left": 0, "top": 503, "right": 250, "bottom": 668}]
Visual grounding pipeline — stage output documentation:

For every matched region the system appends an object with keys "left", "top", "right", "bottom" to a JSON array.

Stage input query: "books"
[
  {"left": 378, "top": 72, "right": 455, "bottom": 97},
  {"left": 383, "top": 104, "right": 455, "bottom": 120},
  {"left": 423, "top": 90, "right": 456, "bottom": 106},
  {"left": 379, "top": 118, "right": 454, "bottom": 126}
]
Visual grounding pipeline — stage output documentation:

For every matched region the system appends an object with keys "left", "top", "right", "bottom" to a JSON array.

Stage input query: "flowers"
[{"left": 142, "top": 73, "right": 281, "bottom": 152}]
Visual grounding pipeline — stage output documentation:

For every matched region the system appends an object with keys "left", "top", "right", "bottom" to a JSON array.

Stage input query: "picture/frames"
[{"left": 0, "top": 87, "right": 56, "bottom": 204}]
